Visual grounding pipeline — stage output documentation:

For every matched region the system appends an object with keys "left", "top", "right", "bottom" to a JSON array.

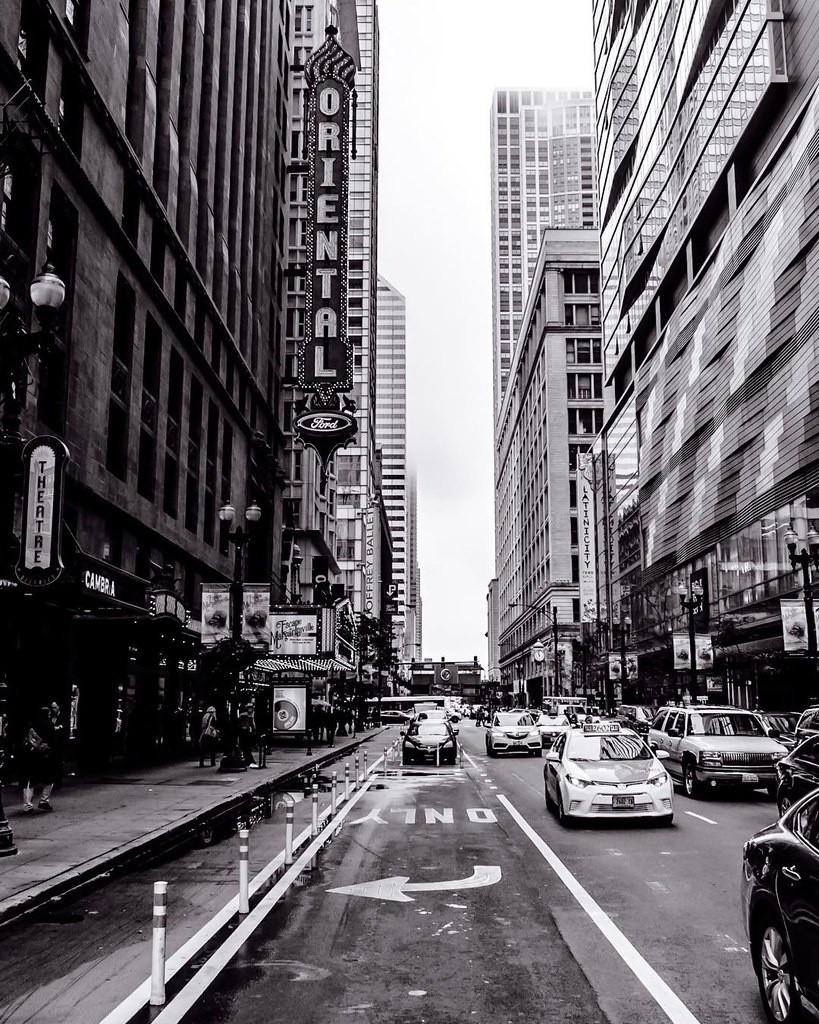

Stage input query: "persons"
[{"left": 18, "top": 691, "right": 768, "bottom": 819}]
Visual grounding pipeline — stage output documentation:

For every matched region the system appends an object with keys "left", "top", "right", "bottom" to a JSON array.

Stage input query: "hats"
[
  {"left": 244, "top": 703, "right": 255, "bottom": 708},
  {"left": 206, "top": 706, "right": 216, "bottom": 712}
]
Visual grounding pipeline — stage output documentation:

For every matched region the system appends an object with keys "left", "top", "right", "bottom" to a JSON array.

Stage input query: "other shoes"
[
  {"left": 23, "top": 802, "right": 34, "bottom": 812},
  {"left": 38, "top": 801, "right": 53, "bottom": 811},
  {"left": 249, "top": 763, "right": 260, "bottom": 768}
]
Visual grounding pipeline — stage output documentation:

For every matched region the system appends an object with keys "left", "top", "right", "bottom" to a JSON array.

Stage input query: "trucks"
[{"left": 414, "top": 703, "right": 462, "bottom": 723}]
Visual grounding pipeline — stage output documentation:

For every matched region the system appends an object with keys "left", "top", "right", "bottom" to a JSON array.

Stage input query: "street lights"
[
  {"left": 784, "top": 525, "right": 819, "bottom": 708},
  {"left": 497, "top": 642, "right": 526, "bottom": 706},
  {"left": 678, "top": 583, "right": 703, "bottom": 701},
  {"left": 612, "top": 617, "right": 632, "bottom": 703},
  {"left": 218, "top": 499, "right": 262, "bottom": 772},
  {"left": 509, "top": 601, "right": 559, "bottom": 695},
  {"left": 0, "top": 262, "right": 67, "bottom": 857}
]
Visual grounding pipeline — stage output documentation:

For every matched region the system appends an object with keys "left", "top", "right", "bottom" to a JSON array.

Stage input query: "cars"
[
  {"left": 534, "top": 713, "right": 571, "bottom": 743},
  {"left": 754, "top": 708, "right": 818, "bottom": 746},
  {"left": 454, "top": 700, "right": 488, "bottom": 719},
  {"left": 543, "top": 723, "right": 675, "bottom": 823},
  {"left": 617, "top": 703, "right": 660, "bottom": 726},
  {"left": 486, "top": 712, "right": 543, "bottom": 758},
  {"left": 648, "top": 708, "right": 790, "bottom": 797},
  {"left": 400, "top": 710, "right": 460, "bottom": 766},
  {"left": 380, "top": 710, "right": 411, "bottom": 724},
  {"left": 775, "top": 733, "right": 819, "bottom": 817},
  {"left": 739, "top": 783, "right": 818, "bottom": 1024}
]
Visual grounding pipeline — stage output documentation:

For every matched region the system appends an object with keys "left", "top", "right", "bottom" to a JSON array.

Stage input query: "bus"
[
  {"left": 542, "top": 696, "right": 588, "bottom": 714},
  {"left": 366, "top": 695, "right": 450, "bottom": 715}
]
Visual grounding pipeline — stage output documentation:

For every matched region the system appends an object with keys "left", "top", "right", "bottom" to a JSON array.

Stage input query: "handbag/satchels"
[
  {"left": 205, "top": 726, "right": 217, "bottom": 738},
  {"left": 22, "top": 729, "right": 49, "bottom": 755}
]
